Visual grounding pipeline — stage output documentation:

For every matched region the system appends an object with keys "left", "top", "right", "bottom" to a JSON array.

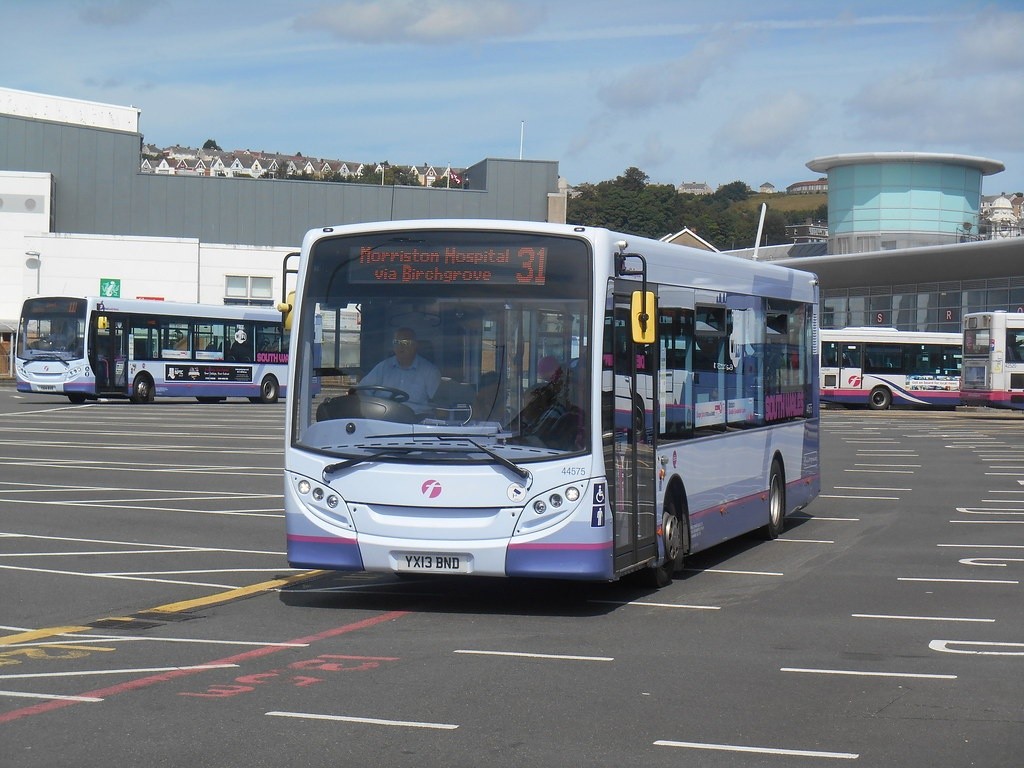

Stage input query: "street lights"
[{"left": 25, "top": 250, "right": 42, "bottom": 297}]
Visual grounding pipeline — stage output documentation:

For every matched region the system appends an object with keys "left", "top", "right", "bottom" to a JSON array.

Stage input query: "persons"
[
  {"left": 471, "top": 313, "right": 803, "bottom": 452},
  {"left": 169, "top": 328, "right": 289, "bottom": 354},
  {"left": 358, "top": 327, "right": 448, "bottom": 424}
]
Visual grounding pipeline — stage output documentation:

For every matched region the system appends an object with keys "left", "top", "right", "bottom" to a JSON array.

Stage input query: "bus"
[
  {"left": 815, "top": 325, "right": 964, "bottom": 413},
  {"left": 13, "top": 293, "right": 323, "bottom": 407},
  {"left": 274, "top": 215, "right": 823, "bottom": 600},
  {"left": 961, "top": 309, "right": 1024, "bottom": 411}
]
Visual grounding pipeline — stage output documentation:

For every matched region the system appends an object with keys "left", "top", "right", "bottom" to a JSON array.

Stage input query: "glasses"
[{"left": 393, "top": 338, "right": 416, "bottom": 345}]
